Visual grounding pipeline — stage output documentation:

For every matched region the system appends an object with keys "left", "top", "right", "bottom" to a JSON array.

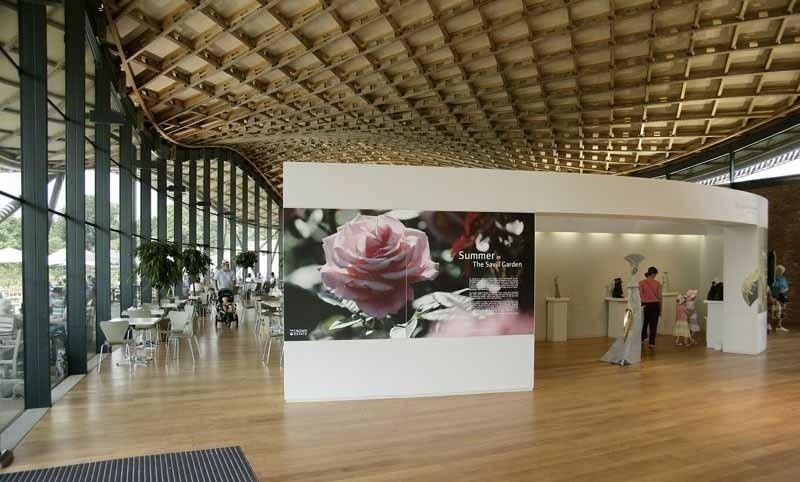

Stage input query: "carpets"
[{"left": 1, "top": 443, "right": 256, "bottom": 482}]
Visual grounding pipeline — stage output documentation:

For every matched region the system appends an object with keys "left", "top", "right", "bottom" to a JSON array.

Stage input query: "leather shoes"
[{"left": 648, "top": 344, "right": 656, "bottom": 347}]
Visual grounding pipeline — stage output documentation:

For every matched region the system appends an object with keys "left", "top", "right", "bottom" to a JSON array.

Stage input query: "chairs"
[{"left": 94, "top": 273, "right": 282, "bottom": 380}]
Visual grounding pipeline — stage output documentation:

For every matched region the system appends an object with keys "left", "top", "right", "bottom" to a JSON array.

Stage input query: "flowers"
[{"left": 317, "top": 215, "right": 441, "bottom": 323}]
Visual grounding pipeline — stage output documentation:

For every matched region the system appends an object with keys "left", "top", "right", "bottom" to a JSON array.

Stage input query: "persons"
[
  {"left": 767, "top": 265, "right": 790, "bottom": 333},
  {"left": 222, "top": 296, "right": 233, "bottom": 313},
  {"left": 246, "top": 272, "right": 276, "bottom": 297},
  {"left": 638, "top": 266, "right": 662, "bottom": 349},
  {"left": 673, "top": 289, "right": 701, "bottom": 347},
  {"left": 214, "top": 260, "right": 236, "bottom": 300}
]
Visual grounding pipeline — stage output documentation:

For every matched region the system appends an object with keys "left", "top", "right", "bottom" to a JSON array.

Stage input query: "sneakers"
[
  {"left": 674, "top": 340, "right": 697, "bottom": 347},
  {"left": 776, "top": 327, "right": 789, "bottom": 331}
]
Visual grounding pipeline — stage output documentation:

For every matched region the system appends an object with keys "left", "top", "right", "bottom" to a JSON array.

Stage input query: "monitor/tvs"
[{"left": 768, "top": 249, "right": 777, "bottom": 286}]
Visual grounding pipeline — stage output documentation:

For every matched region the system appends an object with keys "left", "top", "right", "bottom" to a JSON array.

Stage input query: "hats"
[{"left": 687, "top": 289, "right": 698, "bottom": 300}]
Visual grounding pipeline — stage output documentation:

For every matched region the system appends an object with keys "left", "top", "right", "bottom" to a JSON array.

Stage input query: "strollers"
[{"left": 214, "top": 288, "right": 239, "bottom": 329}]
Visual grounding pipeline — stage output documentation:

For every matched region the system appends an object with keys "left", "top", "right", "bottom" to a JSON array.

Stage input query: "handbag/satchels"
[{"left": 779, "top": 293, "right": 791, "bottom": 302}]
